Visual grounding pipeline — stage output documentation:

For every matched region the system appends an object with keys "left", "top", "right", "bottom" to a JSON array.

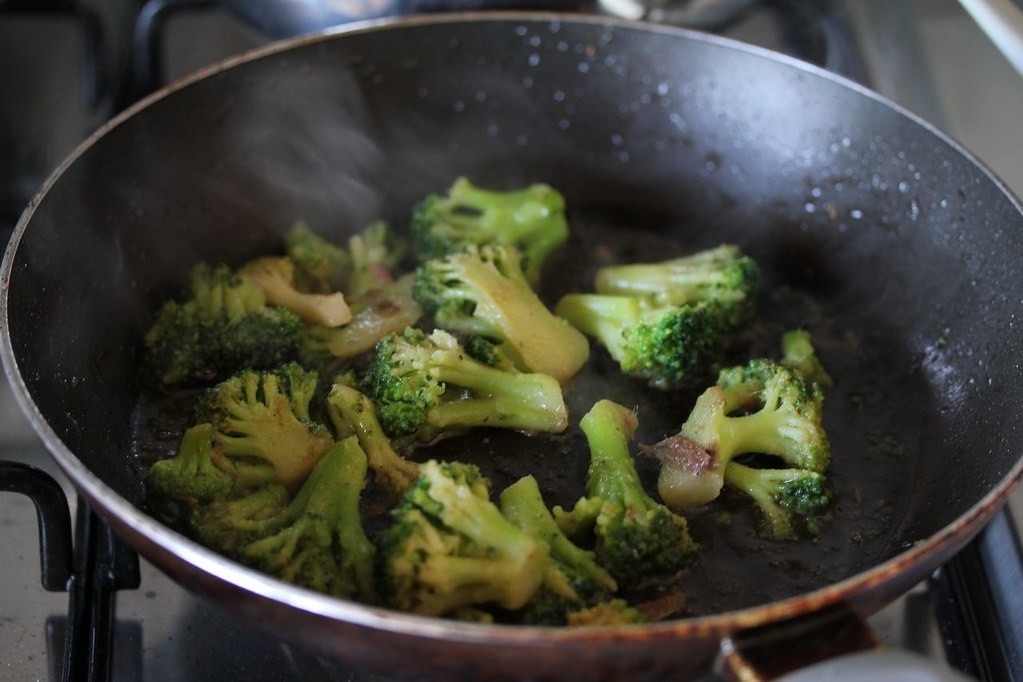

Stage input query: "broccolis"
[{"left": 144, "top": 179, "right": 845, "bottom": 620}]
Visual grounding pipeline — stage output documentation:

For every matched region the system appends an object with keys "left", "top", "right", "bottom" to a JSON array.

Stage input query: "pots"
[{"left": 2, "top": 8, "right": 1021, "bottom": 682}]
